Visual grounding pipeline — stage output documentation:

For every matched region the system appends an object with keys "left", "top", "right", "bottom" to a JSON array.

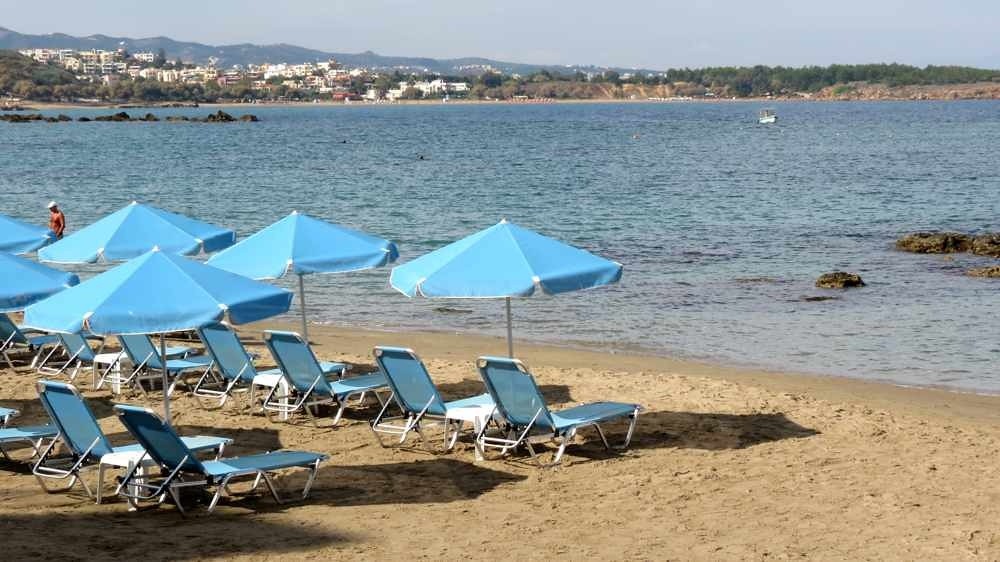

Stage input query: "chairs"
[
  {"left": 53, "top": 331, "right": 192, "bottom": 389},
  {"left": 102, "top": 332, "right": 253, "bottom": 400},
  {"left": 0, "top": 312, "right": 106, "bottom": 373},
  {"left": 31, "top": 379, "right": 235, "bottom": 502},
  {"left": 0, "top": 423, "right": 60, "bottom": 464},
  {"left": 370, "top": 346, "right": 496, "bottom": 452},
  {"left": 114, "top": 405, "right": 330, "bottom": 519},
  {"left": 9, "top": 323, "right": 48, "bottom": 348},
  {"left": 191, "top": 320, "right": 353, "bottom": 410},
  {"left": 0, "top": 407, "right": 21, "bottom": 428},
  {"left": 475, "top": 355, "right": 646, "bottom": 466},
  {"left": 262, "top": 330, "right": 390, "bottom": 427}
]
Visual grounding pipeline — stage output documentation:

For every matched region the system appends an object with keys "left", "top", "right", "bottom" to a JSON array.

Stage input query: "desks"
[
  {"left": 92, "top": 351, "right": 155, "bottom": 394},
  {"left": 96, "top": 449, "right": 155, "bottom": 511},
  {"left": 443, "top": 403, "right": 503, "bottom": 462},
  {"left": 250, "top": 368, "right": 290, "bottom": 420}
]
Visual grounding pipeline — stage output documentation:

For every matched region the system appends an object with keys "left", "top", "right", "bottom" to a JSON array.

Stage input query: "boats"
[{"left": 759, "top": 108, "right": 777, "bottom": 123}]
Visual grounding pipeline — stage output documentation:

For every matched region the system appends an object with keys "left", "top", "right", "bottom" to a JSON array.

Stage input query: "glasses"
[{"left": 49, "top": 207, "right": 54, "bottom": 209}]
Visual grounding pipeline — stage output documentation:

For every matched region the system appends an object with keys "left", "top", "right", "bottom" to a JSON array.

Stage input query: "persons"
[{"left": 48, "top": 201, "right": 65, "bottom": 240}]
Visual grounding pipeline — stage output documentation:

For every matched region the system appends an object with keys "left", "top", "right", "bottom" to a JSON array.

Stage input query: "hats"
[{"left": 47, "top": 201, "right": 57, "bottom": 209}]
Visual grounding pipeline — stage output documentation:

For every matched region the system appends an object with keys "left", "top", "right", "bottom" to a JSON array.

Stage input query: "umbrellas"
[
  {"left": 22, "top": 244, "right": 295, "bottom": 426},
  {"left": 0, "top": 212, "right": 57, "bottom": 250},
  {"left": 389, "top": 217, "right": 623, "bottom": 359},
  {"left": 38, "top": 199, "right": 236, "bottom": 265},
  {"left": 0, "top": 250, "right": 80, "bottom": 313},
  {"left": 204, "top": 211, "right": 399, "bottom": 343}
]
